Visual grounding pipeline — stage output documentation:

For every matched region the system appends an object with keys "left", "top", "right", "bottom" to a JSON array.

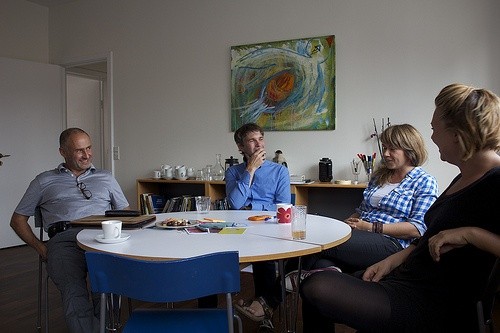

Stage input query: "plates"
[
  {"left": 155, "top": 220, "right": 201, "bottom": 229},
  {"left": 94, "top": 233, "right": 131, "bottom": 244},
  {"left": 162, "top": 177, "right": 173, "bottom": 180},
  {"left": 173, "top": 176, "right": 189, "bottom": 180}
]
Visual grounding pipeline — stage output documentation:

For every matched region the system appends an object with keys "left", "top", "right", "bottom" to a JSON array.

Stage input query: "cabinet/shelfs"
[{"left": 136, "top": 176, "right": 370, "bottom": 224}]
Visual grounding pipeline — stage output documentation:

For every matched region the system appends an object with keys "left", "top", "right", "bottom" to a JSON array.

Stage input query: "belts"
[{"left": 65, "top": 223, "right": 84, "bottom": 228}]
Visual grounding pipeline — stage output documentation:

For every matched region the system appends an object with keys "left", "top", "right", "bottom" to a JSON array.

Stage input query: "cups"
[
  {"left": 276, "top": 203, "right": 293, "bottom": 225},
  {"left": 291, "top": 205, "right": 307, "bottom": 240},
  {"left": 196, "top": 170, "right": 203, "bottom": 180},
  {"left": 161, "top": 164, "right": 195, "bottom": 177},
  {"left": 101, "top": 220, "right": 122, "bottom": 239},
  {"left": 195, "top": 196, "right": 211, "bottom": 214},
  {"left": 152, "top": 171, "right": 161, "bottom": 178}
]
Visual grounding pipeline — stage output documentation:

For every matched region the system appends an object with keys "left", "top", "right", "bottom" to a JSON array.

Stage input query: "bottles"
[
  {"left": 225, "top": 155, "right": 240, "bottom": 172},
  {"left": 211, "top": 154, "right": 225, "bottom": 180},
  {"left": 319, "top": 157, "right": 332, "bottom": 183},
  {"left": 201, "top": 165, "right": 213, "bottom": 181},
  {"left": 273, "top": 150, "right": 284, "bottom": 165}
]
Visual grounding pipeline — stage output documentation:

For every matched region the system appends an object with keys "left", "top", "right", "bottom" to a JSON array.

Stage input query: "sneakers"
[{"left": 281, "top": 266, "right": 341, "bottom": 293}]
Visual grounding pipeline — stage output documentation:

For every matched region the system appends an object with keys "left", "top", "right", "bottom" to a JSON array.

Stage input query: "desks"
[{"left": 75, "top": 208, "right": 352, "bottom": 333}]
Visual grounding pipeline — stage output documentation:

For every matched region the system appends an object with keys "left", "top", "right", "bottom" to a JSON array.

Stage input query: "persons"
[
  {"left": 234, "top": 124, "right": 439, "bottom": 321},
  {"left": 10, "top": 128, "right": 130, "bottom": 333},
  {"left": 280, "top": 83, "right": 500, "bottom": 333},
  {"left": 226, "top": 122, "right": 291, "bottom": 333}
]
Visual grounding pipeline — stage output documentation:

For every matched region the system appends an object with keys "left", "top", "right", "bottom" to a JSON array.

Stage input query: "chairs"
[
  {"left": 85, "top": 250, "right": 241, "bottom": 333},
  {"left": 454, "top": 254, "right": 500, "bottom": 333},
  {"left": 34, "top": 204, "right": 120, "bottom": 333}
]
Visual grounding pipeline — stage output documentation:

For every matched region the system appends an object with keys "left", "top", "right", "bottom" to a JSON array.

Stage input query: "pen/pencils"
[{"left": 353, "top": 159, "right": 357, "bottom": 175}]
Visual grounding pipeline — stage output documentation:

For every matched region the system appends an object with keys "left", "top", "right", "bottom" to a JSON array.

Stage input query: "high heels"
[{"left": 233, "top": 295, "right": 274, "bottom": 333}]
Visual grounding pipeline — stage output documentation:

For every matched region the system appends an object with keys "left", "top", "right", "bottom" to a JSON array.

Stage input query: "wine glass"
[
  {"left": 364, "top": 161, "right": 373, "bottom": 184},
  {"left": 351, "top": 161, "right": 362, "bottom": 184}
]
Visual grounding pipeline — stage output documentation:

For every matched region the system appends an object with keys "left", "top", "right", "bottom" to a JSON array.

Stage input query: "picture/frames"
[{"left": 228, "top": 34, "right": 337, "bottom": 134}]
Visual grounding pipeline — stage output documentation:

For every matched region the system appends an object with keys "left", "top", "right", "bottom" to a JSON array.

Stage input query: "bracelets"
[{"left": 372, "top": 222, "right": 383, "bottom": 233}]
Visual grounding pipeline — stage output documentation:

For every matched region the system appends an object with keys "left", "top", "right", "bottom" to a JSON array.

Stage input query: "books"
[
  {"left": 140, "top": 193, "right": 197, "bottom": 215},
  {"left": 71, "top": 215, "right": 156, "bottom": 229}
]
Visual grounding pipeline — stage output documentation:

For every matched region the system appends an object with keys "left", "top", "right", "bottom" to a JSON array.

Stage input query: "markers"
[
  {"left": 357, "top": 153, "right": 376, "bottom": 174},
  {"left": 265, "top": 216, "right": 277, "bottom": 221}
]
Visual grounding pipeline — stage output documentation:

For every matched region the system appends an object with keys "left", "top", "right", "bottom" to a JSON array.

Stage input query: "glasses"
[{"left": 76, "top": 182, "right": 92, "bottom": 200}]
